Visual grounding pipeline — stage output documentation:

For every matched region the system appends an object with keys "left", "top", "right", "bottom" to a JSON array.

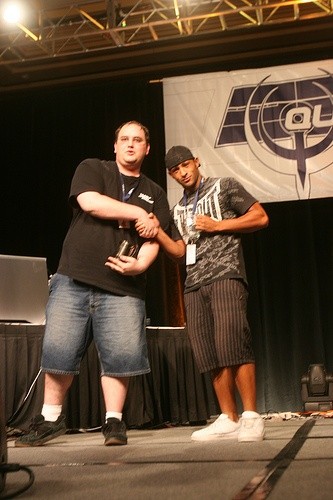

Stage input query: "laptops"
[{"left": 0, "top": 254, "right": 47, "bottom": 321}]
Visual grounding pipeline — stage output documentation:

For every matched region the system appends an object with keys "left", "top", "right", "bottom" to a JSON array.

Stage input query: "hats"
[{"left": 165, "top": 145, "right": 194, "bottom": 172}]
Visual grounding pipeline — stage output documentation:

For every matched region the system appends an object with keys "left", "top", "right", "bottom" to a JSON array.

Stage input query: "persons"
[
  {"left": 136, "top": 146, "right": 269, "bottom": 448},
  {"left": 13, "top": 121, "right": 169, "bottom": 448}
]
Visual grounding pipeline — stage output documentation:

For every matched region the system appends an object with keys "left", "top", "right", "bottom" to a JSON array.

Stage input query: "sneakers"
[
  {"left": 101, "top": 417, "right": 128, "bottom": 445},
  {"left": 236, "top": 414, "right": 266, "bottom": 442},
  {"left": 191, "top": 414, "right": 238, "bottom": 441},
  {"left": 14, "top": 412, "right": 68, "bottom": 447}
]
